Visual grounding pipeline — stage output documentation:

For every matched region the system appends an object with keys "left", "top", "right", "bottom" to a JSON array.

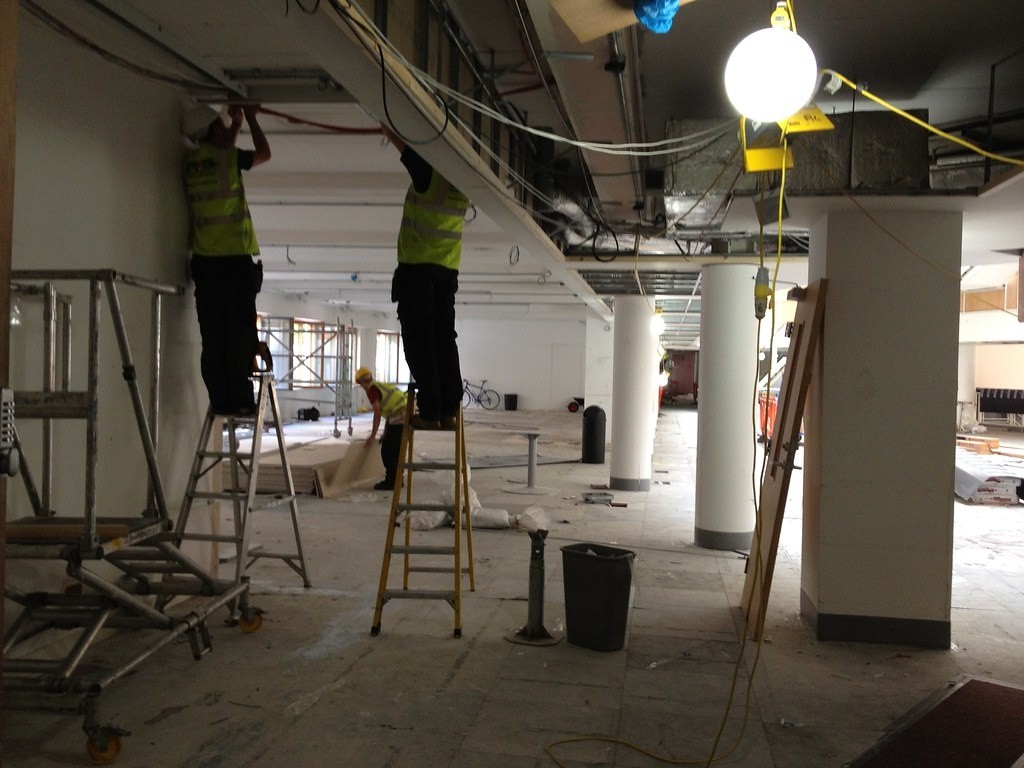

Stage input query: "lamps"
[{"left": 725, "top": 28, "right": 817, "bottom": 122}]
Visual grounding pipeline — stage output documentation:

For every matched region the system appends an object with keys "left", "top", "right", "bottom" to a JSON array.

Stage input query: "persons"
[
  {"left": 183, "top": 98, "right": 273, "bottom": 417},
  {"left": 355, "top": 367, "right": 408, "bottom": 491},
  {"left": 378, "top": 120, "right": 470, "bottom": 428}
]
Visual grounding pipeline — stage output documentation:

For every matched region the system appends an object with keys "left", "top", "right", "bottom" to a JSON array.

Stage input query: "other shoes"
[
  {"left": 410, "top": 415, "right": 441, "bottom": 430},
  {"left": 374, "top": 476, "right": 394, "bottom": 490},
  {"left": 442, "top": 414, "right": 456, "bottom": 429},
  {"left": 212, "top": 402, "right": 256, "bottom": 415}
]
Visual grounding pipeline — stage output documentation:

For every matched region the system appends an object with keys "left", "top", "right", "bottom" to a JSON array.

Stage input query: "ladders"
[
  {"left": 153, "top": 339, "right": 313, "bottom": 626},
  {"left": 369, "top": 383, "right": 475, "bottom": 640}
]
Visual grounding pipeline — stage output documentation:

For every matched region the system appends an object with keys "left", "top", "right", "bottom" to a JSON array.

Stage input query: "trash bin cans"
[
  {"left": 582, "top": 405, "right": 606, "bottom": 464},
  {"left": 559, "top": 540, "right": 637, "bottom": 654},
  {"left": 504, "top": 394, "right": 518, "bottom": 411}
]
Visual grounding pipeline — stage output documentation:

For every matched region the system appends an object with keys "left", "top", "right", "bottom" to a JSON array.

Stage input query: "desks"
[{"left": 498, "top": 429, "right": 561, "bottom": 496}]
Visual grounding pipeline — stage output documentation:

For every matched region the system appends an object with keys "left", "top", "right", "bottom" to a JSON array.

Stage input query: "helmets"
[
  {"left": 182, "top": 104, "right": 219, "bottom": 135},
  {"left": 355, "top": 368, "right": 370, "bottom": 384}
]
